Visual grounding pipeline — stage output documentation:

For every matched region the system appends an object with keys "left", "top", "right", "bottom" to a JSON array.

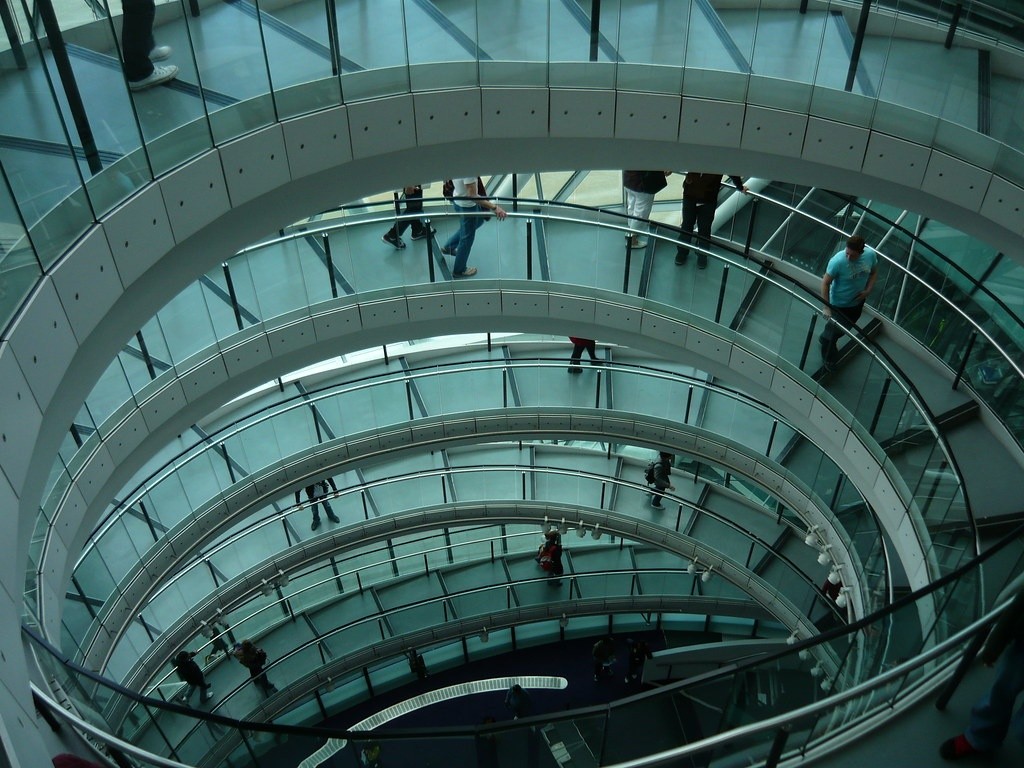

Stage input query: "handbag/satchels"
[{"left": 540, "top": 557, "right": 554, "bottom": 571}]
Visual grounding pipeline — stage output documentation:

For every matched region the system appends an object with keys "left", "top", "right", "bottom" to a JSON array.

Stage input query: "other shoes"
[
  {"left": 548, "top": 581, "right": 563, "bottom": 587},
  {"left": 312, "top": 521, "right": 319, "bottom": 531},
  {"left": 453, "top": 267, "right": 477, "bottom": 278},
  {"left": 626, "top": 241, "right": 647, "bottom": 249},
  {"left": 819, "top": 336, "right": 840, "bottom": 373},
  {"left": 698, "top": 254, "right": 707, "bottom": 269},
  {"left": 183, "top": 696, "right": 189, "bottom": 702},
  {"left": 207, "top": 692, "right": 214, "bottom": 699},
  {"left": 268, "top": 684, "right": 274, "bottom": 689},
  {"left": 592, "top": 361, "right": 601, "bottom": 365},
  {"left": 568, "top": 367, "right": 584, "bottom": 373},
  {"left": 331, "top": 515, "right": 340, "bottom": 523},
  {"left": 651, "top": 504, "right": 665, "bottom": 510},
  {"left": 940, "top": 735, "right": 976, "bottom": 761},
  {"left": 675, "top": 252, "right": 687, "bottom": 264},
  {"left": 441, "top": 247, "right": 456, "bottom": 256}
]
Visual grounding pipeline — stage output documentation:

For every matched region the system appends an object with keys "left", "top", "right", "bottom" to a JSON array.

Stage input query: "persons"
[
  {"left": 200, "top": 623, "right": 273, "bottom": 689},
  {"left": 941, "top": 590, "right": 1024, "bottom": 761},
  {"left": 622, "top": 170, "right": 672, "bottom": 248},
  {"left": 172, "top": 649, "right": 214, "bottom": 706},
  {"left": 675, "top": 172, "right": 750, "bottom": 268},
  {"left": 567, "top": 336, "right": 604, "bottom": 373},
  {"left": 643, "top": 451, "right": 675, "bottom": 510},
  {"left": 505, "top": 684, "right": 531, "bottom": 721},
  {"left": 361, "top": 738, "right": 383, "bottom": 768},
  {"left": 592, "top": 636, "right": 616, "bottom": 682},
  {"left": 382, "top": 183, "right": 437, "bottom": 249},
  {"left": 535, "top": 526, "right": 563, "bottom": 587},
  {"left": 819, "top": 235, "right": 878, "bottom": 365},
  {"left": 821, "top": 569, "right": 840, "bottom": 600},
  {"left": 295, "top": 477, "right": 339, "bottom": 531},
  {"left": 624, "top": 640, "right": 653, "bottom": 683},
  {"left": 119, "top": 0, "right": 179, "bottom": 91},
  {"left": 474, "top": 716, "right": 500, "bottom": 768},
  {"left": 440, "top": 176, "right": 508, "bottom": 279},
  {"left": 409, "top": 650, "right": 427, "bottom": 678}
]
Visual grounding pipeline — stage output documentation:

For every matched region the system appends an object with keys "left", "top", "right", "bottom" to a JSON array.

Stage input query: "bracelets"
[{"left": 492, "top": 206, "right": 497, "bottom": 210}]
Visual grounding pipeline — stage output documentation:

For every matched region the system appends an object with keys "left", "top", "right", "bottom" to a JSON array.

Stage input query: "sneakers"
[
  {"left": 382, "top": 234, "right": 406, "bottom": 250},
  {"left": 148, "top": 46, "right": 171, "bottom": 62},
  {"left": 129, "top": 65, "right": 178, "bottom": 91},
  {"left": 411, "top": 227, "right": 437, "bottom": 240}
]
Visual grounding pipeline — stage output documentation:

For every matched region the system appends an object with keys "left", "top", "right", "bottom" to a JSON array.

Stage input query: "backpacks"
[{"left": 644, "top": 462, "right": 665, "bottom": 484}]
